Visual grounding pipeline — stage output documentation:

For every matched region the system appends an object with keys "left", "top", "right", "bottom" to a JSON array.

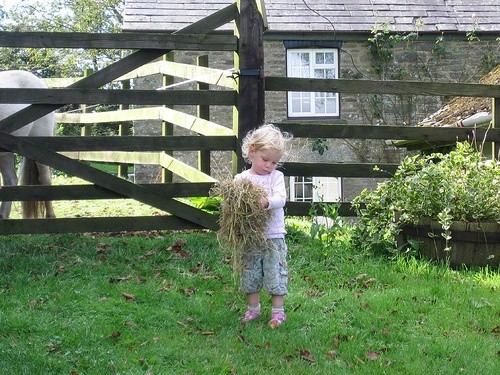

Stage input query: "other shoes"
[
  {"left": 241, "top": 303, "right": 260, "bottom": 323},
  {"left": 268, "top": 308, "right": 286, "bottom": 329}
]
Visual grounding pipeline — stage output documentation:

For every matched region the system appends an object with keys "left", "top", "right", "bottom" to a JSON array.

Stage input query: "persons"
[{"left": 232, "top": 123, "right": 288, "bottom": 329}]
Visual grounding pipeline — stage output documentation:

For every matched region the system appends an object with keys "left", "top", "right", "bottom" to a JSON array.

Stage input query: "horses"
[{"left": 0, "top": 70, "right": 56, "bottom": 219}]
